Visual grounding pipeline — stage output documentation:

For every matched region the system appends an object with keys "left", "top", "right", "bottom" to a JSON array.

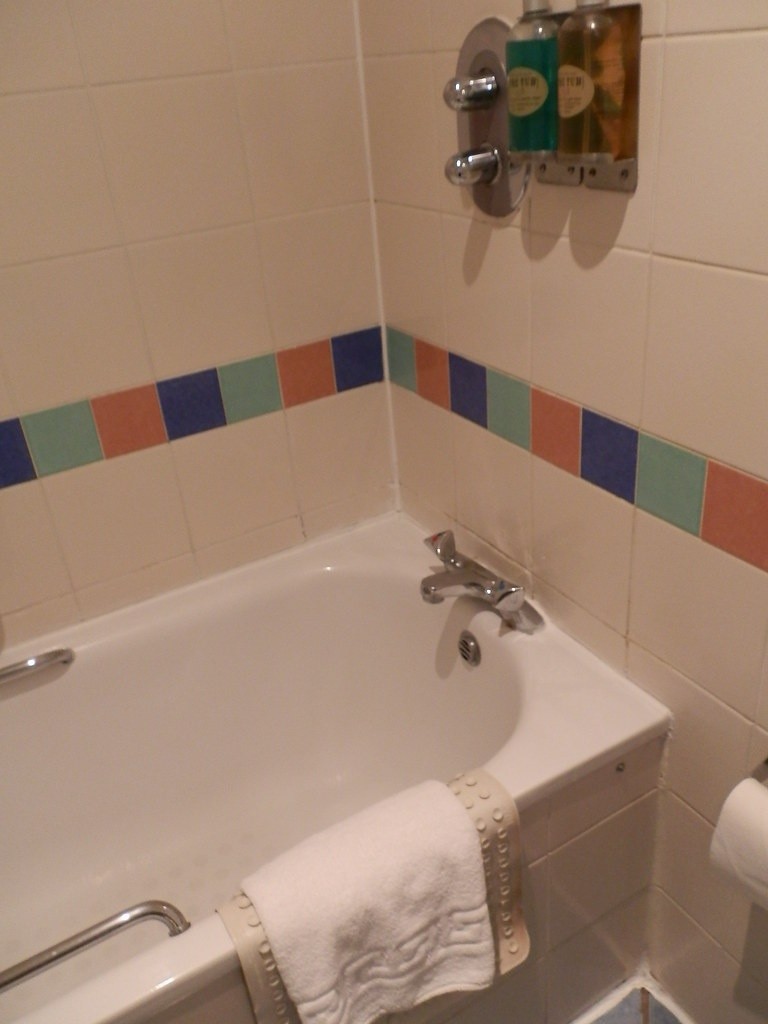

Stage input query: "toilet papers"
[{"left": 706, "top": 777, "right": 768, "bottom": 910}]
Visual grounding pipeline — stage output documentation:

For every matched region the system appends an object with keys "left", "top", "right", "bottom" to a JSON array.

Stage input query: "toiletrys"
[{"left": 499, "top": 0, "right": 625, "bottom": 164}]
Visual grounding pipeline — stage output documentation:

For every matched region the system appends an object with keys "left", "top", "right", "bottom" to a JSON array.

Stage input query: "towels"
[{"left": 242, "top": 780, "right": 496, "bottom": 1024}]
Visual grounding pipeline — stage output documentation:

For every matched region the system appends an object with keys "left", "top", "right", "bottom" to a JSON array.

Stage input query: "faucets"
[{"left": 419, "top": 529, "right": 545, "bottom": 634}]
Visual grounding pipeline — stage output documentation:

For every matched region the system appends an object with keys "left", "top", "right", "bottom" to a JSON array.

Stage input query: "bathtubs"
[{"left": 0, "top": 513, "right": 674, "bottom": 1024}]
